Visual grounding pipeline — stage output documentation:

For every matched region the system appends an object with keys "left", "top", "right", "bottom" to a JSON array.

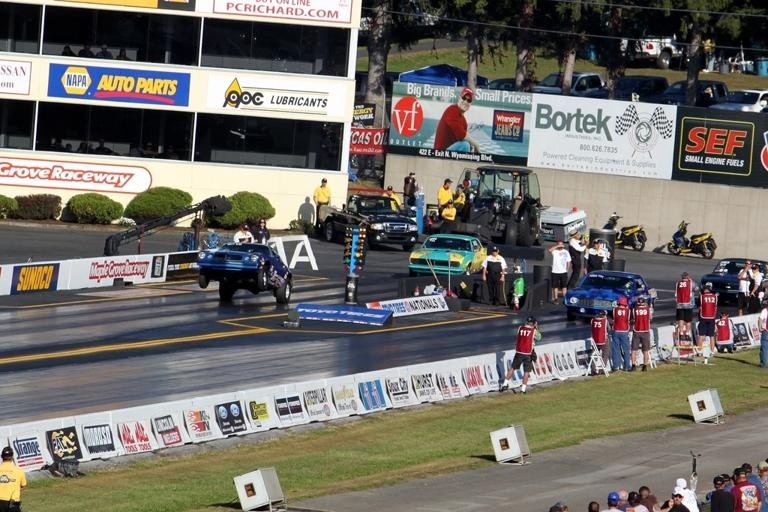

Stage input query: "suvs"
[
  {"left": 659, "top": 78, "right": 729, "bottom": 106},
  {"left": 585, "top": 74, "right": 668, "bottom": 103}
]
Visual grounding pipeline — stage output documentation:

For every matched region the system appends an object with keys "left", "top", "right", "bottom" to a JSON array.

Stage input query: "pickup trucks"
[
  {"left": 531, "top": 69, "right": 607, "bottom": 100},
  {"left": 319, "top": 193, "right": 417, "bottom": 250},
  {"left": 617, "top": 31, "right": 685, "bottom": 70}
]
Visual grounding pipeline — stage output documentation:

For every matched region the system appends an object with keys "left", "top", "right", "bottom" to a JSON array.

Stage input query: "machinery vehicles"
[{"left": 438, "top": 164, "right": 544, "bottom": 247}]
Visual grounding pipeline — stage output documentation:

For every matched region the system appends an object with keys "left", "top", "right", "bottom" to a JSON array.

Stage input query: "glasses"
[{"left": 462, "top": 96, "right": 471, "bottom": 103}]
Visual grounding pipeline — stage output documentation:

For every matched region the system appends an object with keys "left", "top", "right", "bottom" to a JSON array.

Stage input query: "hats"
[
  {"left": 672, "top": 486, "right": 684, "bottom": 497},
  {"left": 713, "top": 461, "right": 767, "bottom": 485},
  {"left": 2, "top": 447, "right": 13, "bottom": 457},
  {"left": 618, "top": 297, "right": 627, "bottom": 305},
  {"left": 461, "top": 88, "right": 473, "bottom": 98},
  {"left": 676, "top": 478, "right": 686, "bottom": 488},
  {"left": 608, "top": 492, "right": 618, "bottom": 500}
]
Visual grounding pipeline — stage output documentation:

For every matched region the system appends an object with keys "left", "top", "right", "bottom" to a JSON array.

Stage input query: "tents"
[{"left": 398, "top": 63, "right": 489, "bottom": 89}]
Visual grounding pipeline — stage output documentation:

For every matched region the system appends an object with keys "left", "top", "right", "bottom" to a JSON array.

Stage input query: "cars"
[
  {"left": 406, "top": 233, "right": 488, "bottom": 279},
  {"left": 196, "top": 243, "right": 292, "bottom": 305},
  {"left": 708, "top": 88, "right": 767, "bottom": 113},
  {"left": 483, "top": 77, "right": 515, "bottom": 91},
  {"left": 695, "top": 258, "right": 767, "bottom": 306},
  {"left": 561, "top": 270, "right": 654, "bottom": 323}
]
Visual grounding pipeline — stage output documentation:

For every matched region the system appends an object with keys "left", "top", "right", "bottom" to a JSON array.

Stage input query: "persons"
[
  {"left": 61, "top": 40, "right": 77, "bottom": 57},
  {"left": 65, "top": 142, "right": 75, "bottom": 151},
  {"left": 96, "top": 43, "right": 113, "bottom": 59},
  {"left": 548, "top": 228, "right": 615, "bottom": 305},
  {"left": 0, "top": 445, "right": 28, "bottom": 512},
  {"left": 46, "top": 136, "right": 65, "bottom": 152},
  {"left": 138, "top": 142, "right": 159, "bottom": 158},
  {"left": 433, "top": 87, "right": 480, "bottom": 153},
  {"left": 704, "top": 37, "right": 712, "bottom": 70},
  {"left": 252, "top": 218, "right": 271, "bottom": 244},
  {"left": 729, "top": 57, "right": 741, "bottom": 73},
  {"left": 544, "top": 458, "right": 768, "bottom": 512},
  {"left": 114, "top": 47, "right": 133, "bottom": 60},
  {"left": 481, "top": 246, "right": 508, "bottom": 306},
  {"left": 178, "top": 219, "right": 204, "bottom": 251},
  {"left": 498, "top": 317, "right": 542, "bottom": 395},
  {"left": 95, "top": 138, "right": 119, "bottom": 155},
  {"left": 77, "top": 40, "right": 96, "bottom": 59},
  {"left": 377, "top": 170, "right": 466, "bottom": 233},
  {"left": 590, "top": 296, "right": 651, "bottom": 375},
  {"left": 77, "top": 141, "right": 88, "bottom": 153},
  {"left": 674, "top": 258, "right": 768, "bottom": 368},
  {"left": 232, "top": 221, "right": 255, "bottom": 246},
  {"left": 313, "top": 177, "right": 331, "bottom": 236}
]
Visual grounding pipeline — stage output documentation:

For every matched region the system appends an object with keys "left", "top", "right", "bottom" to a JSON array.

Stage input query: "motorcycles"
[
  {"left": 668, "top": 221, "right": 715, "bottom": 260},
  {"left": 602, "top": 212, "right": 645, "bottom": 252}
]
